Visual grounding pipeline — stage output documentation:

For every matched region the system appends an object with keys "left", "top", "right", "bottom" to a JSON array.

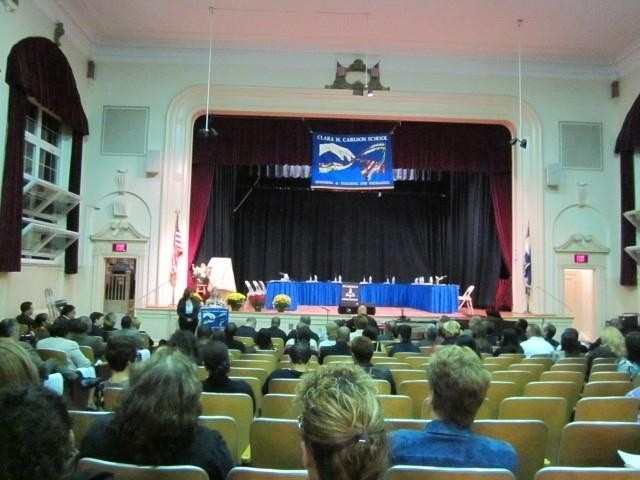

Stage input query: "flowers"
[
  {"left": 272, "top": 294, "right": 293, "bottom": 305},
  {"left": 190, "top": 262, "right": 211, "bottom": 281},
  {"left": 227, "top": 293, "right": 246, "bottom": 305},
  {"left": 251, "top": 293, "right": 270, "bottom": 306}
]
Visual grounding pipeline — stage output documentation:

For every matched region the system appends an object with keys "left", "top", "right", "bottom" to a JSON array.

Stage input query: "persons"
[
  {"left": 0, "top": 293, "right": 640, "bottom": 404},
  {"left": 76, "top": 342, "right": 238, "bottom": 480},
  {"left": 285, "top": 358, "right": 398, "bottom": 480},
  {"left": 379, "top": 343, "right": 521, "bottom": 475},
  {"left": 1, "top": 380, "right": 81, "bottom": 479}
]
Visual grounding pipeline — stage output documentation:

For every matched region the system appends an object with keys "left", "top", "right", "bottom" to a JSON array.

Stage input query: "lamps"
[
  {"left": 354, "top": 12, "right": 376, "bottom": 102},
  {"left": 198, "top": 4, "right": 220, "bottom": 140},
  {"left": 508, "top": 17, "right": 529, "bottom": 150}
]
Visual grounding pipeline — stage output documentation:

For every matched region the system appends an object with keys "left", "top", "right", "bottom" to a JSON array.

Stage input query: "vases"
[
  {"left": 278, "top": 307, "right": 285, "bottom": 312},
  {"left": 196, "top": 277, "right": 208, "bottom": 284},
  {"left": 256, "top": 307, "right": 262, "bottom": 312},
  {"left": 231, "top": 306, "right": 242, "bottom": 311}
]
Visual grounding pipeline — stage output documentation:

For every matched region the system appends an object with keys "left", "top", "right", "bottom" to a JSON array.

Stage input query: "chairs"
[
  {"left": 18, "top": 324, "right": 638, "bottom": 419},
  {"left": 260, "top": 281, "right": 268, "bottom": 297},
  {"left": 497, "top": 396, "right": 570, "bottom": 466},
  {"left": 226, "top": 465, "right": 310, "bottom": 478},
  {"left": 554, "top": 421, "right": 640, "bottom": 467},
  {"left": 249, "top": 419, "right": 383, "bottom": 469},
  {"left": 533, "top": 466, "right": 639, "bottom": 478},
  {"left": 75, "top": 456, "right": 209, "bottom": 479},
  {"left": 244, "top": 276, "right": 262, "bottom": 309},
  {"left": 386, "top": 418, "right": 549, "bottom": 479},
  {"left": 66, "top": 409, "right": 115, "bottom": 452},
  {"left": 199, "top": 414, "right": 241, "bottom": 466},
  {"left": 252, "top": 278, "right": 263, "bottom": 296},
  {"left": 198, "top": 391, "right": 254, "bottom": 463},
  {"left": 456, "top": 284, "right": 474, "bottom": 314},
  {"left": 379, "top": 463, "right": 517, "bottom": 479}
]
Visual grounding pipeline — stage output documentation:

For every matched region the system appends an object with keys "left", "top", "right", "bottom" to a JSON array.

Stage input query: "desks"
[
  {"left": 410, "top": 284, "right": 461, "bottom": 314},
  {"left": 297, "top": 281, "right": 409, "bottom": 309},
  {"left": 265, "top": 279, "right": 299, "bottom": 313}
]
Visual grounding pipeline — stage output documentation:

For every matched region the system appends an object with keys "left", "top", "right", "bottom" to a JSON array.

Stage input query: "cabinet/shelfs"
[{"left": 195, "top": 284, "right": 208, "bottom": 300}]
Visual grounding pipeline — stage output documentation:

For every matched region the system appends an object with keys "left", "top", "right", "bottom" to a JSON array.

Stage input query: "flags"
[
  {"left": 522, "top": 219, "right": 533, "bottom": 297},
  {"left": 309, "top": 129, "right": 396, "bottom": 192},
  {"left": 168, "top": 211, "right": 187, "bottom": 288}
]
